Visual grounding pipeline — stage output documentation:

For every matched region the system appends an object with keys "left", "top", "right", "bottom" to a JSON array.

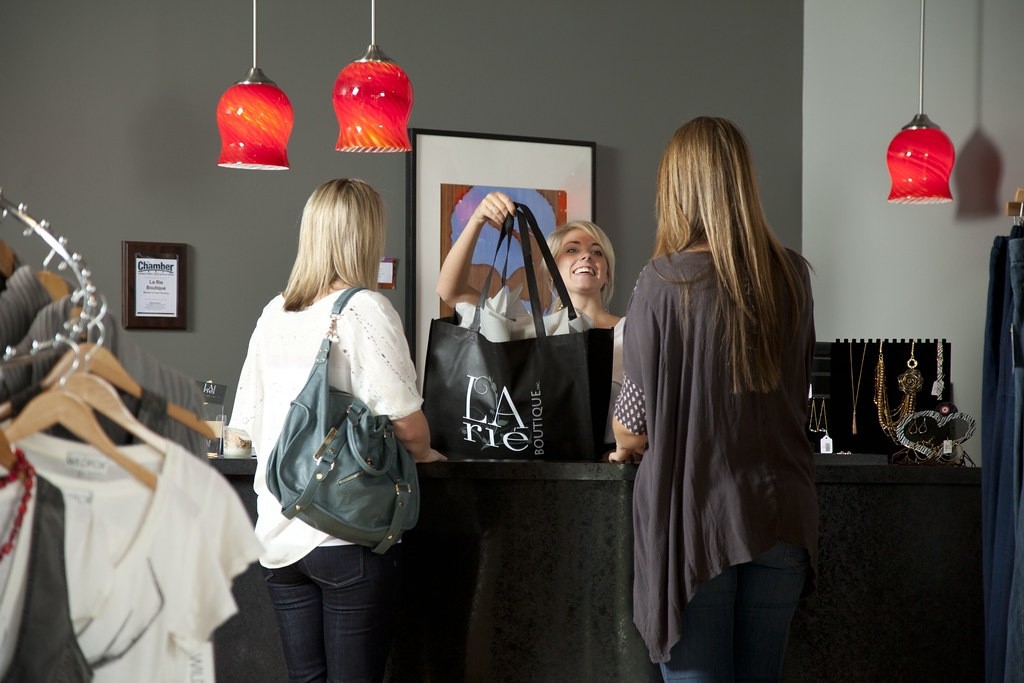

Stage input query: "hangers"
[
  {"left": 1006, "top": 189, "right": 1024, "bottom": 226},
  {"left": 0, "top": 241, "right": 220, "bottom": 499}
]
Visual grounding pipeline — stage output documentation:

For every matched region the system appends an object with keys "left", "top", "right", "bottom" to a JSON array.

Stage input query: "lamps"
[
  {"left": 885, "top": 0, "right": 957, "bottom": 203},
  {"left": 217, "top": 0, "right": 292, "bottom": 173},
  {"left": 331, "top": 0, "right": 415, "bottom": 156}
]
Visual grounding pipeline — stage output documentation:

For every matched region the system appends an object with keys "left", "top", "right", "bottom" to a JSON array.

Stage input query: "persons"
[
  {"left": 607, "top": 116, "right": 819, "bottom": 683},
  {"left": 227, "top": 179, "right": 430, "bottom": 683},
  {"left": 437, "top": 191, "right": 652, "bottom": 683}
]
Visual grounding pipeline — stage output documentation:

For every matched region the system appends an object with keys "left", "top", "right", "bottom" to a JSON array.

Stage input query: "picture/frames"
[
  {"left": 122, "top": 242, "right": 189, "bottom": 331},
  {"left": 406, "top": 128, "right": 598, "bottom": 394}
]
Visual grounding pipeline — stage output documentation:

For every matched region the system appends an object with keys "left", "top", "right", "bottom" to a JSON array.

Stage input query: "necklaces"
[{"left": 850, "top": 341, "right": 943, "bottom": 447}]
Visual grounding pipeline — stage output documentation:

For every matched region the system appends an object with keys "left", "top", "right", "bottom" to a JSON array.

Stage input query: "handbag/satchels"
[
  {"left": 422, "top": 201, "right": 614, "bottom": 463},
  {"left": 266, "top": 287, "right": 421, "bottom": 554}
]
